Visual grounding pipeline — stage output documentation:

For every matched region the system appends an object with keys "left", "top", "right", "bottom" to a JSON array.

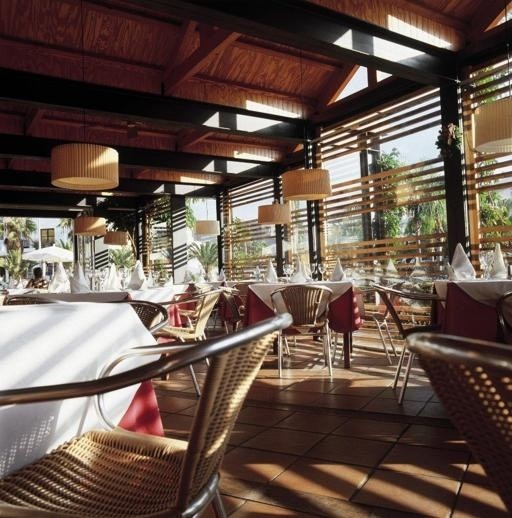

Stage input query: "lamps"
[
  {"left": 49, "top": 1, "right": 121, "bottom": 192},
  {"left": 195, "top": 184, "right": 221, "bottom": 236},
  {"left": 103, "top": 230, "right": 128, "bottom": 245},
  {"left": 258, "top": 199, "right": 292, "bottom": 226},
  {"left": 73, "top": 216, "right": 108, "bottom": 237},
  {"left": 282, "top": 49, "right": 333, "bottom": 201},
  {"left": 472, "top": 0, "right": 512, "bottom": 156}
]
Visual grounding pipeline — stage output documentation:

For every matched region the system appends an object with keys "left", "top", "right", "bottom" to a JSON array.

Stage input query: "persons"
[{"left": 25, "top": 267, "right": 48, "bottom": 289}]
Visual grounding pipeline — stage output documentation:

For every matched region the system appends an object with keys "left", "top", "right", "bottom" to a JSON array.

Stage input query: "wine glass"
[
  {"left": 307, "top": 262, "right": 381, "bottom": 283},
  {"left": 478, "top": 251, "right": 494, "bottom": 281},
  {"left": 282, "top": 264, "right": 294, "bottom": 283},
  {"left": 88, "top": 268, "right": 167, "bottom": 290}
]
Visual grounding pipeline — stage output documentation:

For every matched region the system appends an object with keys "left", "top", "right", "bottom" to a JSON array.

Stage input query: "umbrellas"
[{"left": 21, "top": 244, "right": 74, "bottom": 275}]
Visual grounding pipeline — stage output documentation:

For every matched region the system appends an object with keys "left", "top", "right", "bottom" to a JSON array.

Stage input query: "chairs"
[
  {"left": 406, "top": 333, "right": 512, "bottom": 516},
  {"left": 370, "top": 283, "right": 449, "bottom": 405},
  {"left": 1, "top": 282, "right": 512, "bottom": 381},
  {"left": 1, "top": 312, "right": 292, "bottom": 515}
]
